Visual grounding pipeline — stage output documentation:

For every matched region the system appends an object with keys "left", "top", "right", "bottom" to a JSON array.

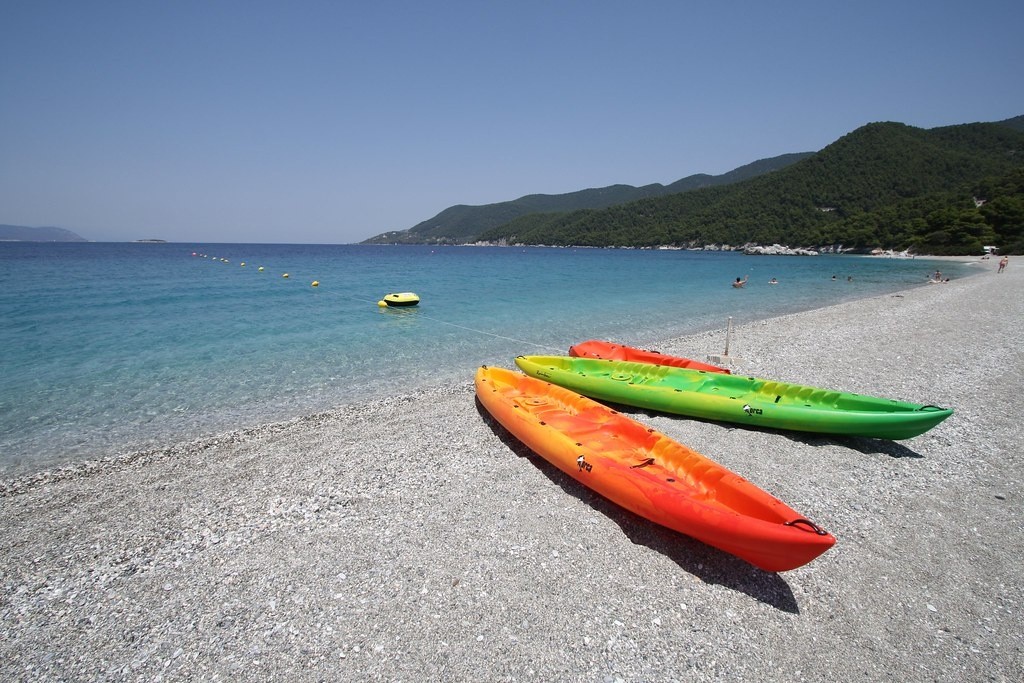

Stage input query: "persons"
[
  {"left": 768, "top": 278, "right": 778, "bottom": 284},
  {"left": 847, "top": 275, "right": 853, "bottom": 282},
  {"left": 832, "top": 276, "right": 836, "bottom": 280},
  {"left": 732, "top": 275, "right": 748, "bottom": 289},
  {"left": 926, "top": 256, "right": 1009, "bottom": 283}
]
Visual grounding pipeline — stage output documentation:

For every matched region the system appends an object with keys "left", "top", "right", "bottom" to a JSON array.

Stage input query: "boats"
[
  {"left": 568, "top": 340, "right": 732, "bottom": 376},
  {"left": 474, "top": 364, "right": 837, "bottom": 572},
  {"left": 514, "top": 355, "right": 955, "bottom": 440},
  {"left": 379, "top": 292, "right": 421, "bottom": 308}
]
